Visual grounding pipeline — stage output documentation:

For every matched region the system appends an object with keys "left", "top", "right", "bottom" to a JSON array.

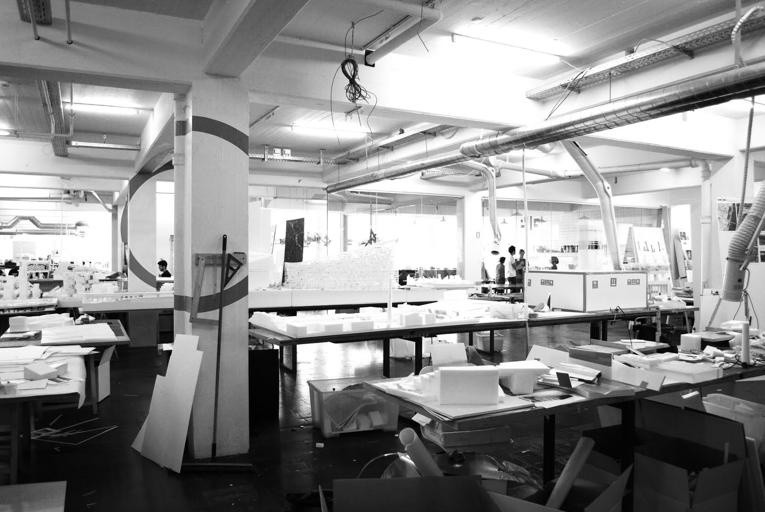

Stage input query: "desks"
[
  {"left": 360, "top": 334, "right": 763, "bottom": 510},
  {"left": 248, "top": 285, "right": 697, "bottom": 377},
  {"left": 0, "top": 287, "right": 175, "bottom": 484}
]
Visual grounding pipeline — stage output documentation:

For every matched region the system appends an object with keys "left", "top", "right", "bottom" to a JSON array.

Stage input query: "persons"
[
  {"left": 481, "top": 244, "right": 525, "bottom": 302},
  {"left": 550, "top": 256, "right": 559, "bottom": 269},
  {"left": 157, "top": 259, "right": 171, "bottom": 277}
]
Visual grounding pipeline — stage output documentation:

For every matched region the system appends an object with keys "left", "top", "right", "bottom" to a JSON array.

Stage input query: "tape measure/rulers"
[
  {"left": 189, "top": 257, "right": 205, "bottom": 323},
  {"left": 223, "top": 253, "right": 243, "bottom": 289}
]
